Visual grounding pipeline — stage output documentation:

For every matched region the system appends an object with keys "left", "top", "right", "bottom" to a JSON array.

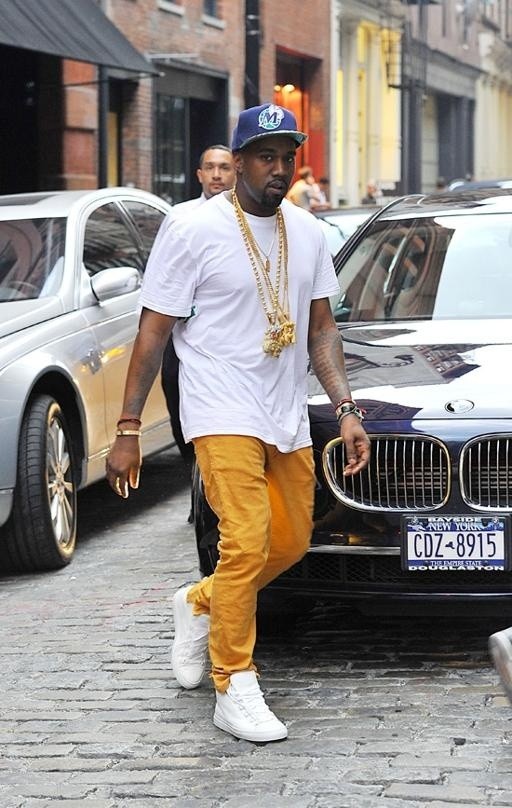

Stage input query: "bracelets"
[
  {"left": 114, "top": 418, "right": 142, "bottom": 427},
  {"left": 115, "top": 429, "right": 141, "bottom": 439},
  {"left": 334, "top": 397, "right": 365, "bottom": 426}
]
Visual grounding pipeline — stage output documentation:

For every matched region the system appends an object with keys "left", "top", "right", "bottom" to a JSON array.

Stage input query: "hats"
[{"left": 231, "top": 103, "right": 309, "bottom": 151}]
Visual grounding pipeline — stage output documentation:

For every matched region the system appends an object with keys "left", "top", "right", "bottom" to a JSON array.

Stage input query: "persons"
[
  {"left": 436, "top": 177, "right": 448, "bottom": 192},
  {"left": 106, "top": 100, "right": 373, "bottom": 745},
  {"left": 286, "top": 166, "right": 378, "bottom": 213},
  {"left": 158, "top": 144, "right": 239, "bottom": 522}
]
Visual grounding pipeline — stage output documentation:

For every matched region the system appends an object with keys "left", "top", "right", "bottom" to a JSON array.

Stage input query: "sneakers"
[
  {"left": 213, "top": 670, "right": 289, "bottom": 743},
  {"left": 171, "top": 586, "right": 210, "bottom": 689}
]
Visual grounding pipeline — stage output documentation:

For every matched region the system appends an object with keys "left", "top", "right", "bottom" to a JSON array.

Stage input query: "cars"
[
  {"left": 0, "top": 186, "right": 180, "bottom": 575},
  {"left": 305, "top": 204, "right": 381, "bottom": 259},
  {"left": 190, "top": 186, "right": 510, "bottom": 621}
]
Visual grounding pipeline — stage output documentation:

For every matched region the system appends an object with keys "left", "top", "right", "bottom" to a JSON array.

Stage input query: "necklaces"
[{"left": 229, "top": 182, "right": 297, "bottom": 363}]
